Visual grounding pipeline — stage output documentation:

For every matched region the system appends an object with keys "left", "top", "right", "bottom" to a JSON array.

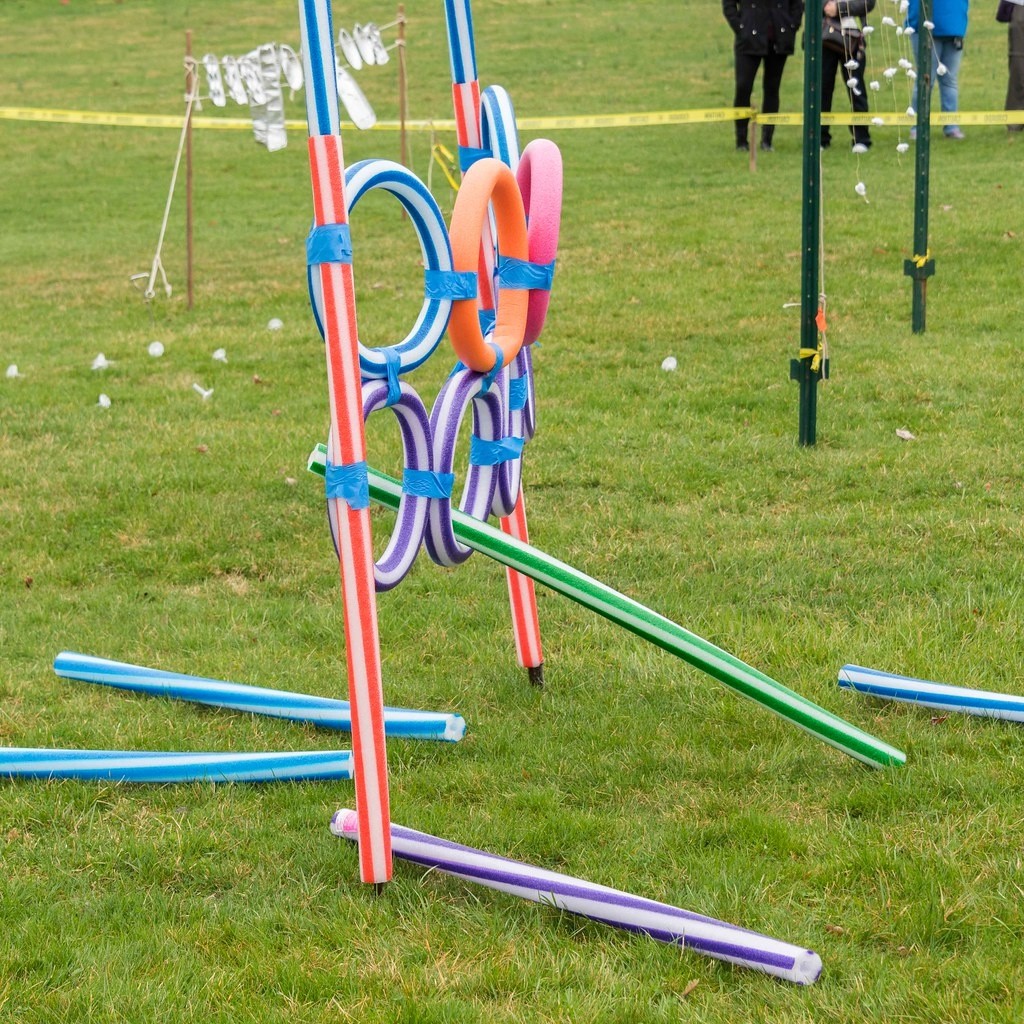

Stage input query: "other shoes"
[
  {"left": 947, "top": 128, "right": 968, "bottom": 139},
  {"left": 909, "top": 132, "right": 920, "bottom": 141},
  {"left": 820, "top": 146, "right": 828, "bottom": 152},
  {"left": 760, "top": 138, "right": 774, "bottom": 152},
  {"left": 852, "top": 143, "right": 868, "bottom": 154},
  {"left": 736, "top": 140, "right": 750, "bottom": 152}
]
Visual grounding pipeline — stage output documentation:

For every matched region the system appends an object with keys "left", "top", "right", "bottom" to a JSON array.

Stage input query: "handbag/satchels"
[
  {"left": 824, "top": 18, "right": 862, "bottom": 56},
  {"left": 996, "top": 0, "right": 1014, "bottom": 23}
]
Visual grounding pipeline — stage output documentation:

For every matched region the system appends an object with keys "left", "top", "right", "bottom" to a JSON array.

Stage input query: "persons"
[
  {"left": 801, "top": 0, "right": 876, "bottom": 153},
  {"left": 903, "top": 0, "right": 969, "bottom": 139},
  {"left": 722, "top": 0, "right": 804, "bottom": 152},
  {"left": 997, "top": 0, "right": 1024, "bottom": 131}
]
